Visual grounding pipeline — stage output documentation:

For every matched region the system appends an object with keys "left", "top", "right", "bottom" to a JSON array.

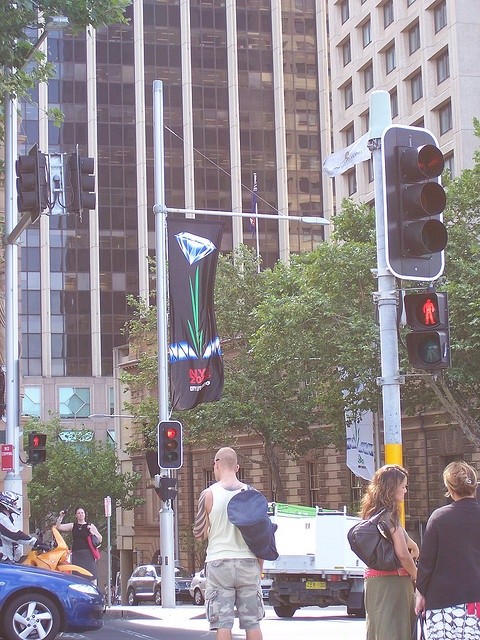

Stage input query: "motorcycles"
[{"left": 18, "top": 524, "right": 94, "bottom": 578}]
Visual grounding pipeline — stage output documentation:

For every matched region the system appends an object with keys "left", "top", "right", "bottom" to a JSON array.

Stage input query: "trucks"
[{"left": 262, "top": 507, "right": 373, "bottom": 618}]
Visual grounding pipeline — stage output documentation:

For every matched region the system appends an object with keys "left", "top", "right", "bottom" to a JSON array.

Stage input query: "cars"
[
  {"left": 190, "top": 567, "right": 275, "bottom": 605},
  {"left": 0, "top": 560, "right": 105, "bottom": 640}
]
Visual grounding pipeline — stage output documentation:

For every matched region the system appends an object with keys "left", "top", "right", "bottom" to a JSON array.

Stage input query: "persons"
[
  {"left": 415, "top": 461, "right": 480, "bottom": 640},
  {"left": 193, "top": 447, "right": 264, "bottom": 640},
  {"left": 55, "top": 507, "right": 102, "bottom": 587},
  {"left": 358, "top": 465, "right": 420, "bottom": 640}
]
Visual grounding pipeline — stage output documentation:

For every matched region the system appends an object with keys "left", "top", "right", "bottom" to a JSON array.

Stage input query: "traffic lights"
[
  {"left": 381, "top": 123, "right": 449, "bottom": 281},
  {"left": 26, "top": 430, "right": 50, "bottom": 468},
  {"left": 402, "top": 290, "right": 450, "bottom": 331},
  {"left": 403, "top": 331, "right": 452, "bottom": 372},
  {"left": 151, "top": 476, "right": 180, "bottom": 503},
  {"left": 13, "top": 141, "right": 49, "bottom": 224},
  {"left": 156, "top": 419, "right": 185, "bottom": 471},
  {"left": 69, "top": 141, "right": 99, "bottom": 218}
]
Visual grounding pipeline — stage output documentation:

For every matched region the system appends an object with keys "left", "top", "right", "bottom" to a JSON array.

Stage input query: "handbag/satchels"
[
  {"left": 347, "top": 507, "right": 401, "bottom": 572},
  {"left": 87, "top": 534, "right": 101, "bottom": 560},
  {"left": 227, "top": 484, "right": 279, "bottom": 561},
  {"left": 89, "top": 523, "right": 100, "bottom": 547}
]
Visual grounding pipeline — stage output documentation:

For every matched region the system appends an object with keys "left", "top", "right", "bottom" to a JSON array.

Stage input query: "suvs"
[{"left": 127, "top": 564, "right": 193, "bottom": 606}]
